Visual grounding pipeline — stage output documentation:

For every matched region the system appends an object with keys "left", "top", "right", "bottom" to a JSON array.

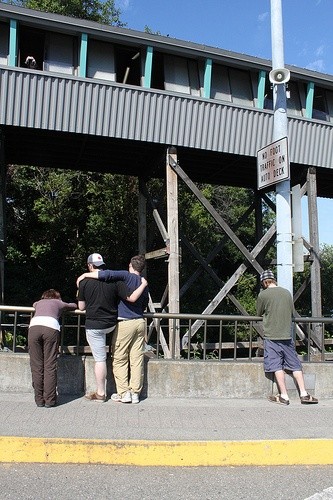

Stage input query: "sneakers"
[
  {"left": 110, "top": 391, "right": 131, "bottom": 402},
  {"left": 130, "top": 393, "right": 139, "bottom": 404}
]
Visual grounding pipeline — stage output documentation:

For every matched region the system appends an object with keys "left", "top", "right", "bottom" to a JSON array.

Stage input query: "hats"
[
  {"left": 260, "top": 269, "right": 274, "bottom": 281},
  {"left": 86, "top": 253, "right": 105, "bottom": 266}
]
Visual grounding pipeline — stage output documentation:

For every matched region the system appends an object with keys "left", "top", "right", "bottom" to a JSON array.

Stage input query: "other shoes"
[
  {"left": 83, "top": 392, "right": 105, "bottom": 402},
  {"left": 300, "top": 392, "right": 318, "bottom": 404},
  {"left": 267, "top": 393, "right": 288, "bottom": 406}
]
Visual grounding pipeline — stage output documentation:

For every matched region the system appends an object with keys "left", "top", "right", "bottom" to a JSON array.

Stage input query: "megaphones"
[{"left": 269, "top": 68, "right": 290, "bottom": 84}]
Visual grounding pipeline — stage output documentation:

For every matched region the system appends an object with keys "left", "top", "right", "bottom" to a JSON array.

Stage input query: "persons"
[
  {"left": 77, "top": 253, "right": 148, "bottom": 402},
  {"left": 28, "top": 289, "right": 77, "bottom": 408},
  {"left": 76, "top": 255, "right": 149, "bottom": 404},
  {"left": 256, "top": 270, "right": 318, "bottom": 405}
]
